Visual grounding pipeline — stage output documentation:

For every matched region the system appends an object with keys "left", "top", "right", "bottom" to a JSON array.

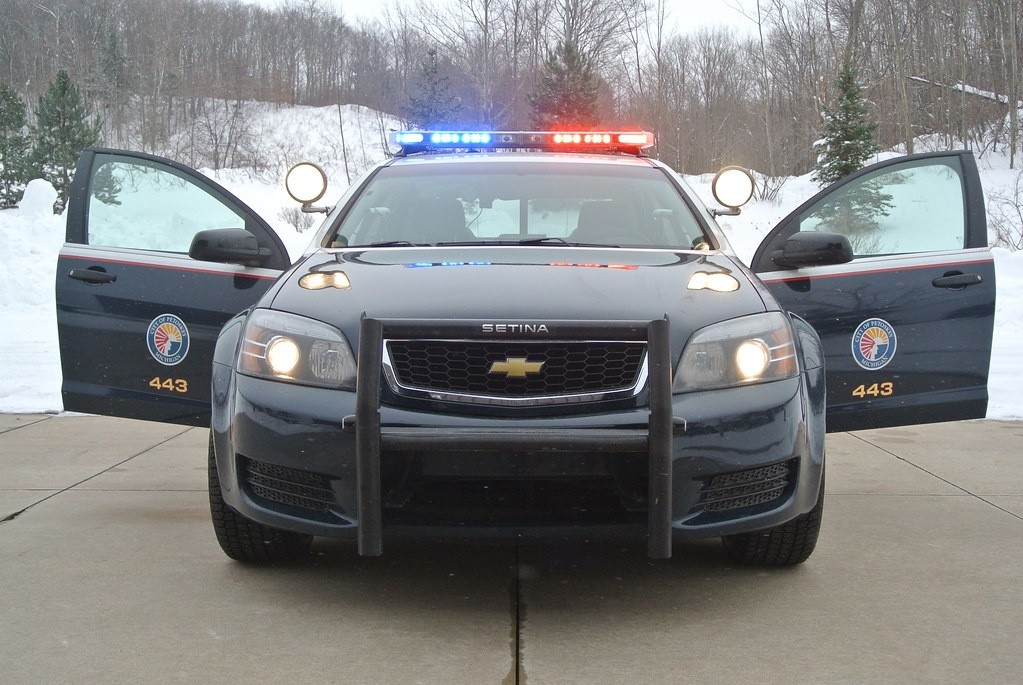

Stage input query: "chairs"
[
  {"left": 409, "top": 198, "right": 477, "bottom": 242},
  {"left": 565, "top": 199, "right": 654, "bottom": 246}
]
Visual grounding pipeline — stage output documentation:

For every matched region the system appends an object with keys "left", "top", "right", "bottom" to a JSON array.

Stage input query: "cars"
[{"left": 55, "top": 131, "right": 994, "bottom": 567}]
